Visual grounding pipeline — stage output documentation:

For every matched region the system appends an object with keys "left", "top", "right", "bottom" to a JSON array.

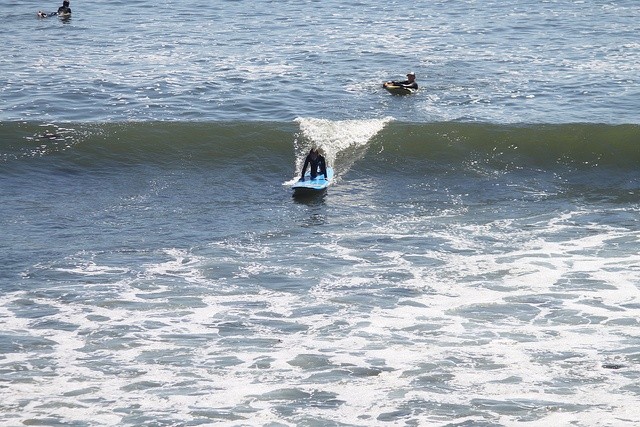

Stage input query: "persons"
[
  {"left": 37, "top": 0, "right": 72, "bottom": 18},
  {"left": 382, "top": 72, "right": 418, "bottom": 90},
  {"left": 298, "top": 148, "right": 329, "bottom": 181}
]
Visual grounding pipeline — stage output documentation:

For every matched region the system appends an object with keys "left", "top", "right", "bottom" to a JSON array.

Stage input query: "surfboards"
[
  {"left": 290, "top": 166, "right": 334, "bottom": 190},
  {"left": 384, "top": 85, "right": 414, "bottom": 95}
]
